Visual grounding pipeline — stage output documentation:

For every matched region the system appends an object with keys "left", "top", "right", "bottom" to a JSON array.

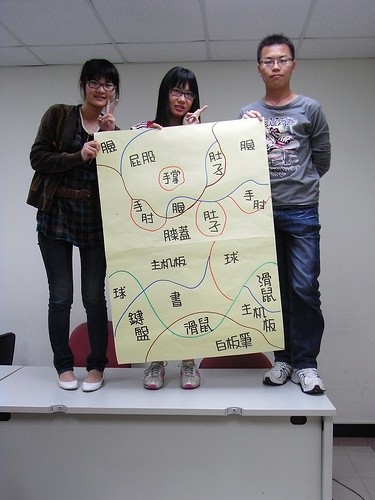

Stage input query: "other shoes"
[
  {"left": 82, "top": 370, "right": 105, "bottom": 392},
  {"left": 55, "top": 371, "right": 78, "bottom": 390}
]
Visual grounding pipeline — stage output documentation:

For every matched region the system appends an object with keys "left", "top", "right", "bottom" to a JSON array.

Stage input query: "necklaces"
[{"left": 264, "top": 92, "right": 292, "bottom": 107}]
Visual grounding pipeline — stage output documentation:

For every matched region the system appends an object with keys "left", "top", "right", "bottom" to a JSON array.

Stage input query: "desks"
[{"left": 0, "top": 365, "right": 336, "bottom": 499}]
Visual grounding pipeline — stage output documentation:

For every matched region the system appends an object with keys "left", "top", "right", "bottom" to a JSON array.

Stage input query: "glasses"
[
  {"left": 260, "top": 57, "right": 293, "bottom": 65},
  {"left": 88, "top": 79, "right": 117, "bottom": 90},
  {"left": 170, "top": 88, "right": 195, "bottom": 100}
]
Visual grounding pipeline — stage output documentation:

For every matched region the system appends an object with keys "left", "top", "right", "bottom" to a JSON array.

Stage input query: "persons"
[
  {"left": 233, "top": 35, "right": 333, "bottom": 397},
  {"left": 127, "top": 66, "right": 208, "bottom": 391},
  {"left": 25, "top": 57, "right": 122, "bottom": 393}
]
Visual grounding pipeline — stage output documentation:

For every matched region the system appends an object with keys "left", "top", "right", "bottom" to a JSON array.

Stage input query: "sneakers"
[
  {"left": 143, "top": 362, "right": 166, "bottom": 390},
  {"left": 179, "top": 362, "right": 200, "bottom": 389},
  {"left": 290, "top": 368, "right": 326, "bottom": 394},
  {"left": 262, "top": 362, "right": 292, "bottom": 385}
]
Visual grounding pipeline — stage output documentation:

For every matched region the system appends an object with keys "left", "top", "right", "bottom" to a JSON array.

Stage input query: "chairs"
[{"left": 69, "top": 321, "right": 132, "bottom": 369}]
